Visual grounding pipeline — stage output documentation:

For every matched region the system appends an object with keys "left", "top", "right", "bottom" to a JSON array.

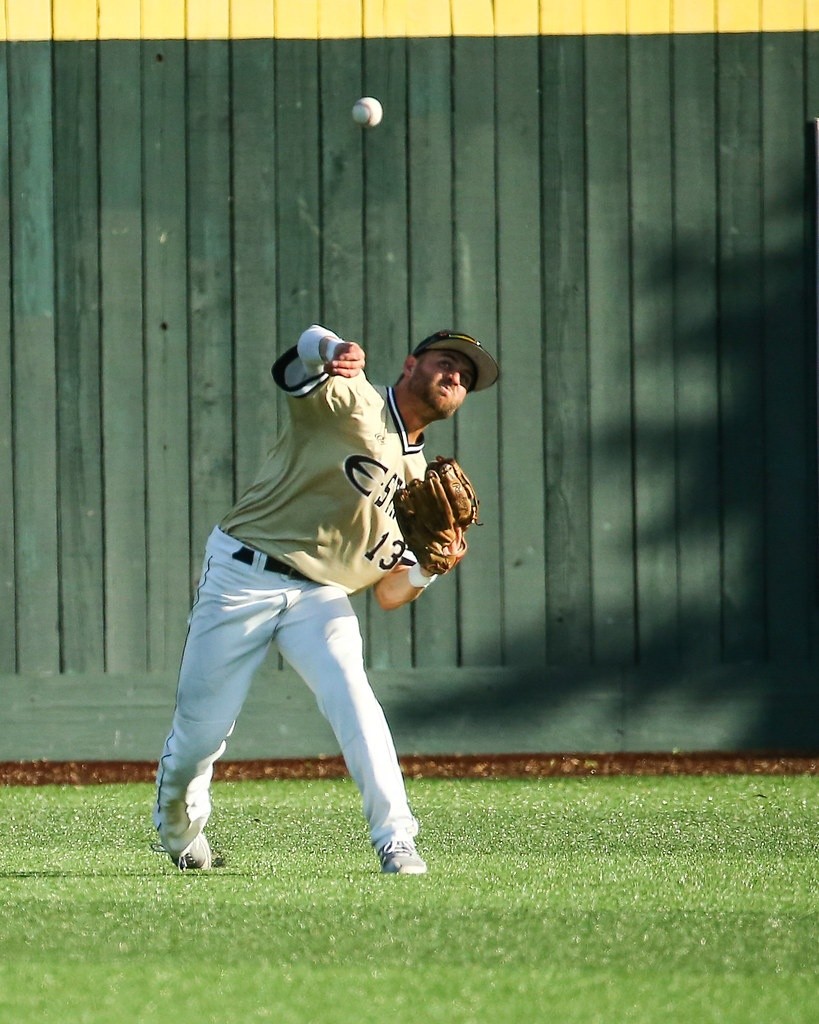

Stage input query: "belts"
[{"left": 233, "top": 546, "right": 312, "bottom": 581}]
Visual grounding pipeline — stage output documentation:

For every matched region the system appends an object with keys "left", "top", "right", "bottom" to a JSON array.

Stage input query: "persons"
[{"left": 153, "top": 325, "right": 500, "bottom": 874}]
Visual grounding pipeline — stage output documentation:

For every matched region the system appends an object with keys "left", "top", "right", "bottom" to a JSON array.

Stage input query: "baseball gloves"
[{"left": 392, "top": 454, "right": 485, "bottom": 577}]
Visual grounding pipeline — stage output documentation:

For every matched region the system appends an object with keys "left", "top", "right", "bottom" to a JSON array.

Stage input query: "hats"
[{"left": 413, "top": 330, "right": 498, "bottom": 392}]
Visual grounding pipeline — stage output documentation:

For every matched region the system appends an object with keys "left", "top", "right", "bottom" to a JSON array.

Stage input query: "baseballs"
[{"left": 351, "top": 95, "right": 384, "bottom": 128}]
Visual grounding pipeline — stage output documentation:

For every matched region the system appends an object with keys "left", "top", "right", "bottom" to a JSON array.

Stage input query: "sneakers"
[
  {"left": 378, "top": 839, "right": 428, "bottom": 875},
  {"left": 164, "top": 830, "right": 211, "bottom": 870}
]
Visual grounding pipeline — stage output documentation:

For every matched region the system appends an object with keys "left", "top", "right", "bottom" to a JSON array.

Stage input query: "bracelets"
[
  {"left": 409, "top": 563, "right": 437, "bottom": 587},
  {"left": 326, "top": 339, "right": 344, "bottom": 362}
]
